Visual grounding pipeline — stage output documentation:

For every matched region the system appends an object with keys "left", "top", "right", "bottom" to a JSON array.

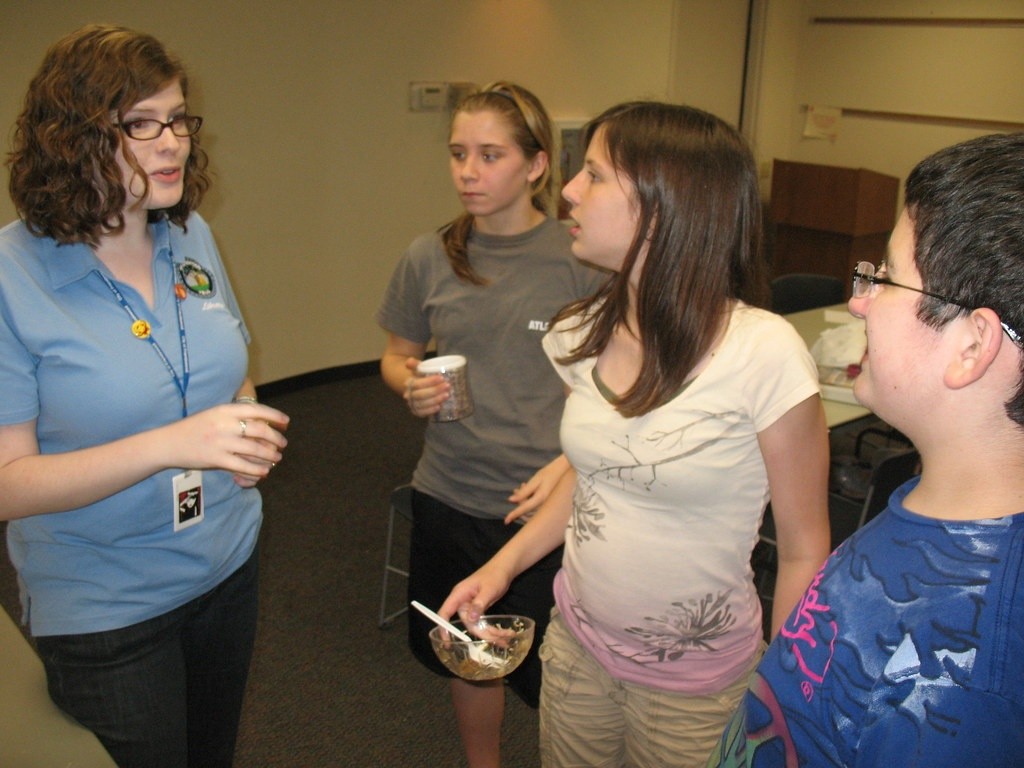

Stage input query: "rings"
[{"left": 239, "top": 420, "right": 247, "bottom": 438}]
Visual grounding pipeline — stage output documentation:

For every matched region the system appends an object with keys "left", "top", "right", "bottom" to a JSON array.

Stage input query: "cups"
[{"left": 416, "top": 355, "right": 476, "bottom": 422}]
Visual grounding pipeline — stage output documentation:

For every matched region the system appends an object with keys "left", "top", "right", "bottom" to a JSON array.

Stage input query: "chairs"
[
  {"left": 768, "top": 274, "right": 840, "bottom": 316},
  {"left": 750, "top": 427, "right": 922, "bottom": 618},
  {"left": 377, "top": 485, "right": 410, "bottom": 632}
]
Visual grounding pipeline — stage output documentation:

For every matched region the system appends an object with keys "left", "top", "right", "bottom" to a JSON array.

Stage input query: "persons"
[
  {"left": 436, "top": 99, "right": 831, "bottom": 767},
  {"left": 0, "top": 23, "right": 291, "bottom": 768},
  {"left": 704, "top": 130, "right": 1024, "bottom": 768},
  {"left": 375, "top": 78, "right": 616, "bottom": 768}
]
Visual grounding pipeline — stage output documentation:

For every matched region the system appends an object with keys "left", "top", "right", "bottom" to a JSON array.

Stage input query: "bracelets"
[{"left": 235, "top": 396, "right": 257, "bottom": 404}]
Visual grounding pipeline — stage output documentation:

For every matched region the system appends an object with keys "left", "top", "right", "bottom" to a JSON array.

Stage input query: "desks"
[{"left": 782, "top": 304, "right": 875, "bottom": 427}]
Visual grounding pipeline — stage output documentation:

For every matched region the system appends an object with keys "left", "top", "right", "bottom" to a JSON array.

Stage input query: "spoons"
[{"left": 411, "top": 599, "right": 509, "bottom": 670}]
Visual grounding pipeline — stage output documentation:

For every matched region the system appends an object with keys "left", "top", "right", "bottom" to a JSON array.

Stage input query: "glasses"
[
  {"left": 852, "top": 261, "right": 1024, "bottom": 350},
  {"left": 112, "top": 116, "right": 203, "bottom": 141}
]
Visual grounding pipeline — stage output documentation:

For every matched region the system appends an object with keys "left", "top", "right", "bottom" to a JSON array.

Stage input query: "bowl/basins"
[{"left": 429, "top": 614, "right": 536, "bottom": 682}]
